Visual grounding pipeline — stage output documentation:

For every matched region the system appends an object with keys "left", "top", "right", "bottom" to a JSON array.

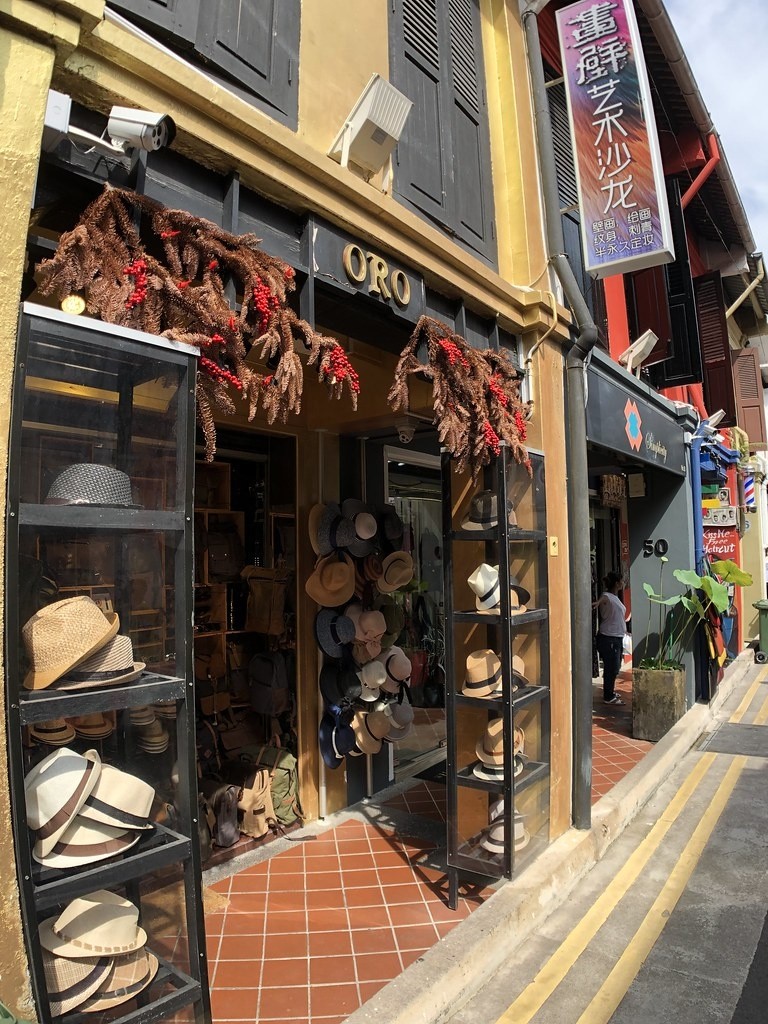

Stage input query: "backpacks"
[{"left": 194, "top": 464, "right": 317, "bottom": 847}]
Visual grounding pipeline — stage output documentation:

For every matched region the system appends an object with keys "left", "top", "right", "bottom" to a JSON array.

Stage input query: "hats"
[
  {"left": 38, "top": 889, "right": 147, "bottom": 958},
  {"left": 22, "top": 595, "right": 120, "bottom": 690},
  {"left": 480, "top": 822, "right": 530, "bottom": 854},
  {"left": 24, "top": 747, "right": 155, "bottom": 868},
  {"left": 28, "top": 699, "right": 177, "bottom": 754},
  {"left": 44, "top": 462, "right": 144, "bottom": 509},
  {"left": 46, "top": 634, "right": 147, "bottom": 690},
  {"left": 461, "top": 649, "right": 529, "bottom": 700},
  {"left": 76, "top": 945, "right": 159, "bottom": 1012},
  {"left": 305, "top": 498, "right": 414, "bottom": 770},
  {"left": 41, "top": 946, "right": 115, "bottom": 1018},
  {"left": 460, "top": 490, "right": 513, "bottom": 531},
  {"left": 473, "top": 717, "right": 528, "bottom": 781},
  {"left": 466, "top": 563, "right": 530, "bottom": 616},
  {"left": 480, "top": 799, "right": 526, "bottom": 833}
]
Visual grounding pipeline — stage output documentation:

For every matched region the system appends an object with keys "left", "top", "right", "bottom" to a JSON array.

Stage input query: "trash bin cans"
[{"left": 751, "top": 598, "right": 768, "bottom": 664}]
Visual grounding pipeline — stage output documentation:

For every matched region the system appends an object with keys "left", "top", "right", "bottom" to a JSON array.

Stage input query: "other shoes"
[
  {"left": 604, "top": 697, "right": 626, "bottom": 705},
  {"left": 613, "top": 692, "right": 621, "bottom": 697}
]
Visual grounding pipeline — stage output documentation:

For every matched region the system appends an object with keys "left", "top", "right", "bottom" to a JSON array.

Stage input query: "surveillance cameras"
[
  {"left": 396, "top": 423, "right": 416, "bottom": 443},
  {"left": 107, "top": 105, "right": 176, "bottom": 152},
  {"left": 703, "top": 425, "right": 721, "bottom": 436}
]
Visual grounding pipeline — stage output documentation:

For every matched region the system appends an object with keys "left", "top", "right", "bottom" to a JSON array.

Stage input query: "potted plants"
[{"left": 632, "top": 556, "right": 753, "bottom": 742}]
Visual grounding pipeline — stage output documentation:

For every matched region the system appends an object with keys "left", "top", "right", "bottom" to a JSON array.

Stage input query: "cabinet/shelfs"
[
  {"left": 4, "top": 437, "right": 297, "bottom": 1024},
  {"left": 440, "top": 442, "right": 554, "bottom": 911}
]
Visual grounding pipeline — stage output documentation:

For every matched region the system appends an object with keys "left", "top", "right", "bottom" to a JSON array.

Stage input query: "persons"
[{"left": 591, "top": 570, "right": 626, "bottom": 705}]
